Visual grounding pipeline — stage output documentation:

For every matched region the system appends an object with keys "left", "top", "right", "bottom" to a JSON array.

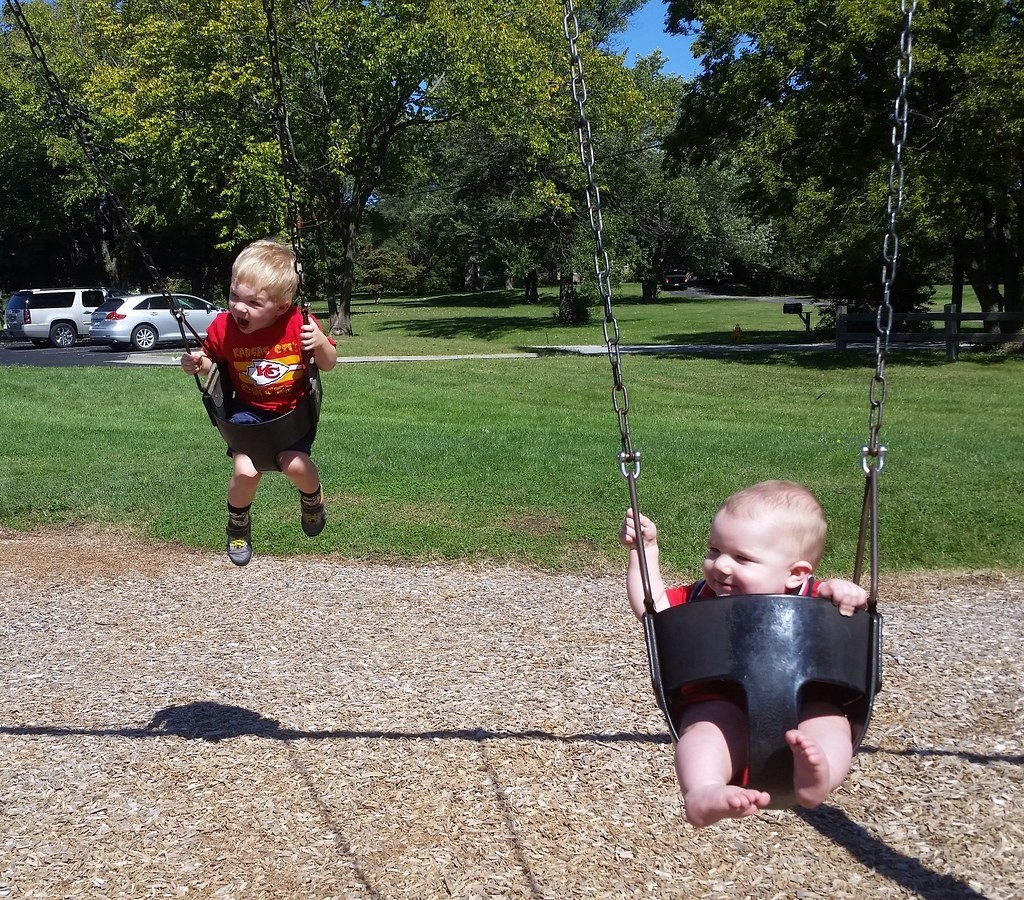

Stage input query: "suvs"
[{"left": 2, "top": 286, "right": 124, "bottom": 349}]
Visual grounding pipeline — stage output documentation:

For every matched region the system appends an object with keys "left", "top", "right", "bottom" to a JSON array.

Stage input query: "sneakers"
[
  {"left": 226, "top": 513, "right": 252, "bottom": 566},
  {"left": 300, "top": 483, "right": 327, "bottom": 537}
]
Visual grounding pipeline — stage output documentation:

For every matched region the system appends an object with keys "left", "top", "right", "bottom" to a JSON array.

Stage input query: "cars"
[
  {"left": 87, "top": 293, "right": 229, "bottom": 352},
  {"left": 661, "top": 269, "right": 690, "bottom": 290}
]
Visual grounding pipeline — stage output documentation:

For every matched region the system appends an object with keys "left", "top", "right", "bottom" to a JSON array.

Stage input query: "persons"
[
  {"left": 618, "top": 480, "right": 868, "bottom": 828},
  {"left": 182, "top": 242, "right": 336, "bottom": 566}
]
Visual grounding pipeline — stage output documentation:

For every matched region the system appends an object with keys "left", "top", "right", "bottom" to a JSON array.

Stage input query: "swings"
[
  {"left": 7, "top": 0, "right": 324, "bottom": 473},
  {"left": 563, "top": 0, "right": 919, "bottom": 810}
]
